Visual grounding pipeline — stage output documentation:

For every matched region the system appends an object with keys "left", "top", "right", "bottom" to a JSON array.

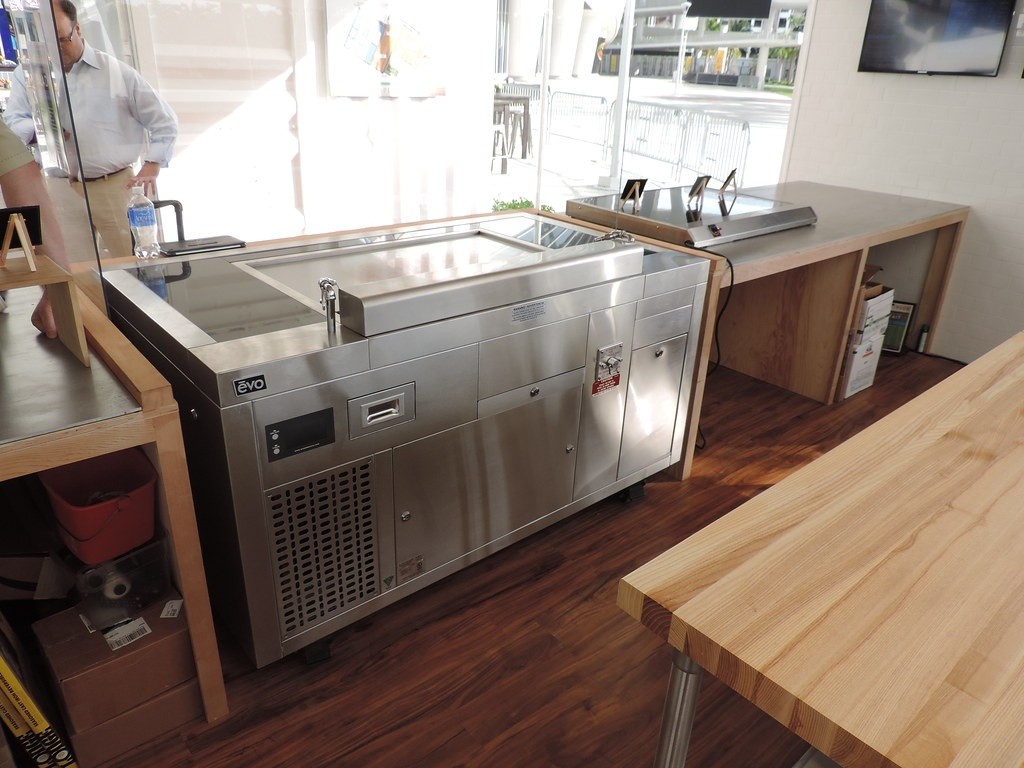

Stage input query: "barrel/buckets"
[{"left": 38, "top": 446, "right": 158, "bottom": 566}]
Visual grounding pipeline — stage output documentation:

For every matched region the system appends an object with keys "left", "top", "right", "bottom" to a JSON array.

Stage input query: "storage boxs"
[
  {"left": 854, "top": 287, "right": 896, "bottom": 345},
  {"left": 860, "top": 264, "right": 885, "bottom": 301},
  {"left": 31, "top": 584, "right": 197, "bottom": 735},
  {"left": 54, "top": 674, "right": 205, "bottom": 768},
  {"left": 843, "top": 334, "right": 885, "bottom": 400}
]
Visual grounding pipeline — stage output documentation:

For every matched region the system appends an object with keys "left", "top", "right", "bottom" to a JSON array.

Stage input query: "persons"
[
  {"left": 0, "top": 118, "right": 71, "bottom": 338},
  {"left": 2, "top": 0, "right": 178, "bottom": 256}
]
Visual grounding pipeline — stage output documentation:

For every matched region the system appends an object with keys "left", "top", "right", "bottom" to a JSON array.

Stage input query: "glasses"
[{"left": 59, "top": 22, "right": 77, "bottom": 46}]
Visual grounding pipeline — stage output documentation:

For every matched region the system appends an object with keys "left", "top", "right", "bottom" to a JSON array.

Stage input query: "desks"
[
  {"left": 615, "top": 330, "right": 1024, "bottom": 768},
  {"left": 556, "top": 180, "right": 970, "bottom": 482},
  {"left": 0, "top": 284, "right": 230, "bottom": 768}
]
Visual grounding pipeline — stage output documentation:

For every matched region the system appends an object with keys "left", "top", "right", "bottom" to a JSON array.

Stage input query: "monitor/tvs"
[{"left": 857, "top": 0, "right": 1017, "bottom": 77}]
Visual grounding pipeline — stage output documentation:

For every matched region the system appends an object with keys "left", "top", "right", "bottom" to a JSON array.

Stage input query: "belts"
[{"left": 65, "top": 167, "right": 128, "bottom": 182}]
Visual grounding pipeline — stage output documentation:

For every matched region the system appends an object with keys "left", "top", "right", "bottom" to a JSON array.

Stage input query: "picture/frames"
[{"left": 882, "top": 300, "right": 918, "bottom": 355}]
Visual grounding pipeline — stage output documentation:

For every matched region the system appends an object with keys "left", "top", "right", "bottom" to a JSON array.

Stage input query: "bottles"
[{"left": 127, "top": 185, "right": 160, "bottom": 259}]
